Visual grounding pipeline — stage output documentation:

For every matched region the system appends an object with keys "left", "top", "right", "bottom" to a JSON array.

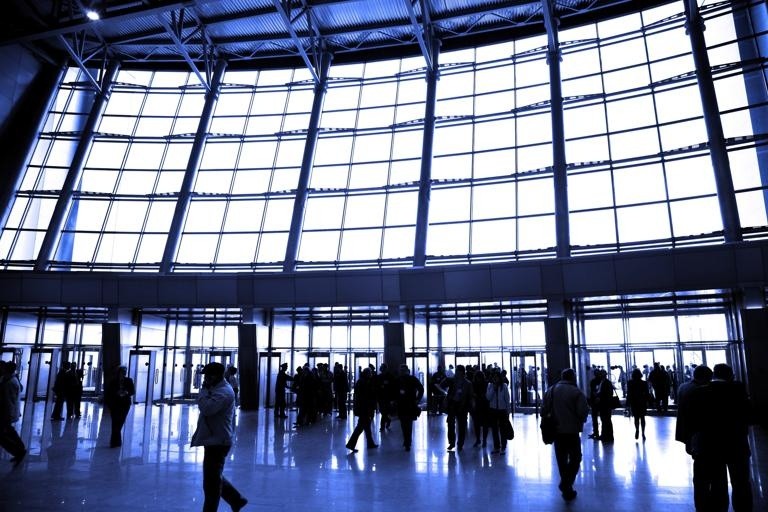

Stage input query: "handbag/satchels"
[
  {"left": 612, "top": 395, "right": 621, "bottom": 410},
  {"left": 540, "top": 411, "right": 555, "bottom": 446},
  {"left": 505, "top": 420, "right": 515, "bottom": 440}
]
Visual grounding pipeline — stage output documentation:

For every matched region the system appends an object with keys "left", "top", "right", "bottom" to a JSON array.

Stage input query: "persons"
[
  {"left": 68, "top": 361, "right": 82, "bottom": 419},
  {"left": 0, "top": 358, "right": 29, "bottom": 465},
  {"left": 188, "top": 360, "right": 249, "bottom": 512},
  {"left": 710, "top": 360, "right": 763, "bottom": 511},
  {"left": 106, "top": 364, "right": 136, "bottom": 449},
  {"left": 274, "top": 357, "right": 705, "bottom": 458},
  {"left": 674, "top": 363, "right": 714, "bottom": 510},
  {"left": 49, "top": 360, "right": 71, "bottom": 422},
  {"left": 224, "top": 363, "right": 240, "bottom": 433},
  {"left": 539, "top": 367, "right": 591, "bottom": 503}
]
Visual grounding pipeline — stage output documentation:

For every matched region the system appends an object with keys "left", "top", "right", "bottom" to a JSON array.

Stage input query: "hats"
[
  {"left": 280, "top": 363, "right": 288, "bottom": 367},
  {"left": 200, "top": 361, "right": 226, "bottom": 376}
]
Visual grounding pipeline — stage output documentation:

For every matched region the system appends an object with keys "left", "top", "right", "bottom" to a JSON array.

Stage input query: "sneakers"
[
  {"left": 111, "top": 438, "right": 122, "bottom": 446},
  {"left": 293, "top": 414, "right": 348, "bottom": 428},
  {"left": 346, "top": 444, "right": 358, "bottom": 453},
  {"left": 403, "top": 441, "right": 411, "bottom": 451},
  {"left": 457, "top": 443, "right": 463, "bottom": 451},
  {"left": 8, "top": 450, "right": 27, "bottom": 462},
  {"left": 635, "top": 431, "right": 639, "bottom": 439},
  {"left": 231, "top": 497, "right": 249, "bottom": 512},
  {"left": 642, "top": 434, "right": 646, "bottom": 440},
  {"left": 380, "top": 426, "right": 392, "bottom": 431},
  {"left": 558, "top": 482, "right": 578, "bottom": 502},
  {"left": 274, "top": 413, "right": 288, "bottom": 419},
  {"left": 588, "top": 432, "right": 614, "bottom": 442},
  {"left": 499, "top": 450, "right": 506, "bottom": 455},
  {"left": 368, "top": 443, "right": 379, "bottom": 449},
  {"left": 447, "top": 442, "right": 455, "bottom": 450},
  {"left": 491, "top": 448, "right": 500, "bottom": 454},
  {"left": 50, "top": 413, "right": 80, "bottom": 422}
]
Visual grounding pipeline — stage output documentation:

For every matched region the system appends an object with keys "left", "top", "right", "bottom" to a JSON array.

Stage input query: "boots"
[
  {"left": 482, "top": 435, "right": 488, "bottom": 448},
  {"left": 473, "top": 435, "right": 481, "bottom": 447}
]
